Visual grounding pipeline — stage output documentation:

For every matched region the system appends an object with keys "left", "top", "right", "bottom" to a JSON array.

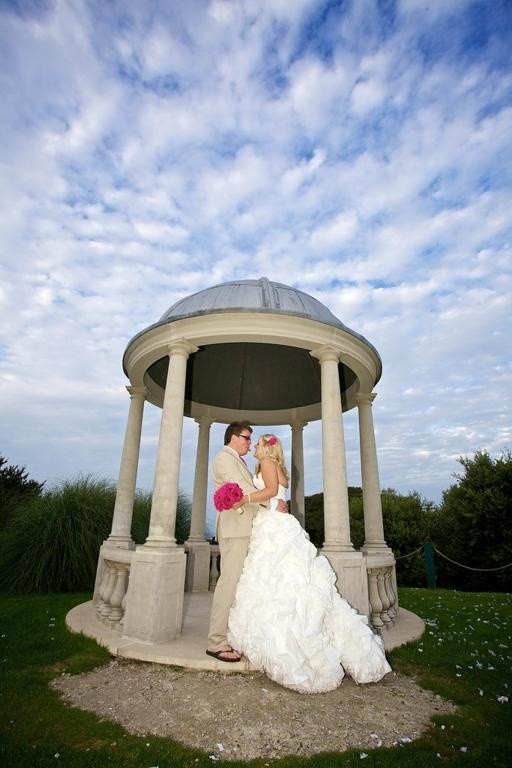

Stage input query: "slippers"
[{"left": 206, "top": 646, "right": 243, "bottom": 662}]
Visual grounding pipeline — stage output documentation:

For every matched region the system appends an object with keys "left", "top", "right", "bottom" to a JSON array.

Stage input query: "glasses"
[{"left": 238, "top": 435, "right": 251, "bottom": 441}]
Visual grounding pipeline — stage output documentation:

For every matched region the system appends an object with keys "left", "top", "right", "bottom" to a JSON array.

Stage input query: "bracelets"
[{"left": 247, "top": 494, "right": 250, "bottom": 504}]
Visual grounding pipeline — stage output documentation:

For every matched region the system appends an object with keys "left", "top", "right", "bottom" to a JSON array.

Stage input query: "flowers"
[
  {"left": 268, "top": 436, "right": 277, "bottom": 445},
  {"left": 212, "top": 483, "right": 245, "bottom": 515}
]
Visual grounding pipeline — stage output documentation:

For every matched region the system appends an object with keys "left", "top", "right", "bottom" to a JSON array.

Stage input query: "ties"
[{"left": 239, "top": 456, "right": 247, "bottom": 465}]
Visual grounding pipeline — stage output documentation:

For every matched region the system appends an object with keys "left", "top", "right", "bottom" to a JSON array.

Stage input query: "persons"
[
  {"left": 206, "top": 422, "right": 289, "bottom": 662},
  {"left": 226, "top": 434, "right": 392, "bottom": 694}
]
